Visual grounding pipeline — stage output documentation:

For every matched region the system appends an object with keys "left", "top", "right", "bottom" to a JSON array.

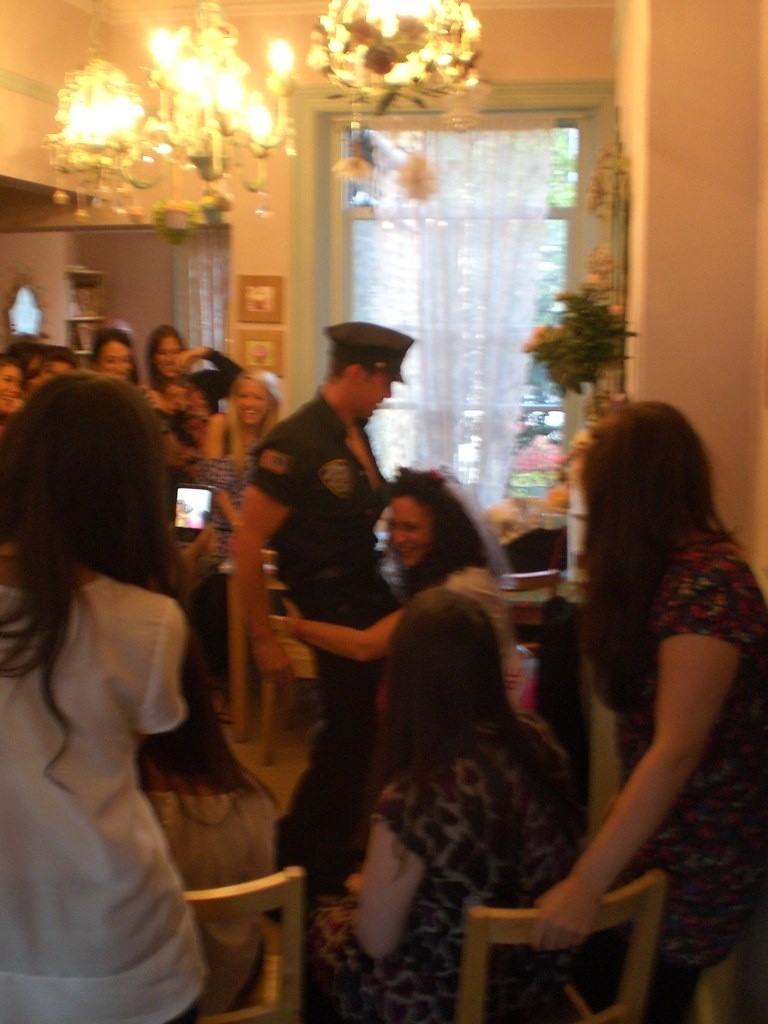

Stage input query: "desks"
[{"left": 219, "top": 557, "right": 291, "bottom": 743}]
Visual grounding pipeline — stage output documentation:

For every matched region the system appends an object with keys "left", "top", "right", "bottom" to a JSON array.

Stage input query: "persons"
[
  {"left": 535, "top": 402, "right": 768, "bottom": 1024},
  {"left": 0, "top": 322, "right": 575, "bottom": 1024}
]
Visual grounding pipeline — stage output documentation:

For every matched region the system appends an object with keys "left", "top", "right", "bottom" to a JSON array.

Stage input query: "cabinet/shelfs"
[{"left": 61, "top": 264, "right": 105, "bottom": 373}]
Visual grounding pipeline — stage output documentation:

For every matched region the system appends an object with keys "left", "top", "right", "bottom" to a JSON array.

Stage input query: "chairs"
[
  {"left": 454, "top": 868, "right": 669, "bottom": 1023},
  {"left": 182, "top": 866, "right": 302, "bottom": 1024}
]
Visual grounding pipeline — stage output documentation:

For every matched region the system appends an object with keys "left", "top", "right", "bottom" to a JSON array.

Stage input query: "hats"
[{"left": 322, "top": 322, "right": 413, "bottom": 382}]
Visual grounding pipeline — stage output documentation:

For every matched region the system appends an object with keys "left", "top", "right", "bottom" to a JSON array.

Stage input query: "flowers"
[{"left": 520, "top": 269, "right": 626, "bottom": 391}]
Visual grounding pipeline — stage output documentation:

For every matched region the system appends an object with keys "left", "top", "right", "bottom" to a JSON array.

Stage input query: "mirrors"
[{"left": 4, "top": 273, "right": 48, "bottom": 344}]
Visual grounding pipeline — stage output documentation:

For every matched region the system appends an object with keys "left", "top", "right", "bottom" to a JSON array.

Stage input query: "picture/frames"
[
  {"left": 234, "top": 273, "right": 284, "bottom": 322},
  {"left": 234, "top": 329, "right": 285, "bottom": 377}
]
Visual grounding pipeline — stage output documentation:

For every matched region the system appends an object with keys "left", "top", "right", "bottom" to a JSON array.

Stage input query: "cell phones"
[{"left": 174, "top": 483, "right": 215, "bottom": 541}]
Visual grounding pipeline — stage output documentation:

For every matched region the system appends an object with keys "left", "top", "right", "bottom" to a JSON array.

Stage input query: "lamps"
[
  {"left": 38, "top": 0, "right": 299, "bottom": 247},
  {"left": 306, "top": 0, "right": 482, "bottom": 111}
]
